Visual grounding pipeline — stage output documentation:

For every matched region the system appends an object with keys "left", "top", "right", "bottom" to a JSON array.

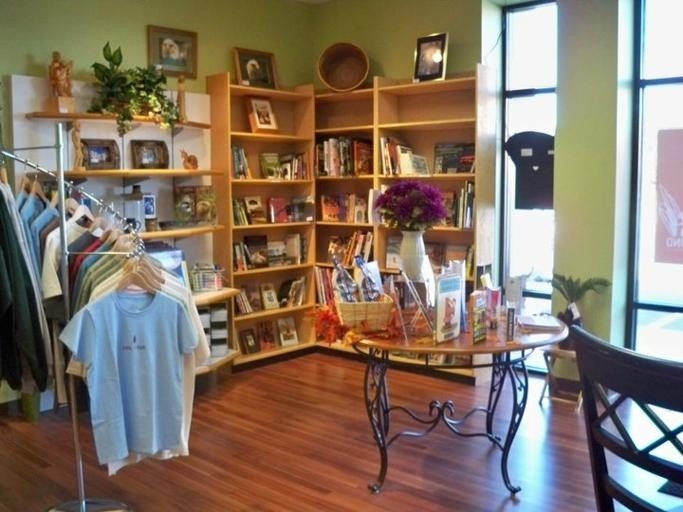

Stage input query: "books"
[
  {"left": 432, "top": 277, "right": 562, "bottom": 345},
  {"left": 232, "top": 145, "right": 314, "bottom": 355},
  {"left": 390, "top": 350, "right": 473, "bottom": 365},
  {"left": 367, "top": 137, "right": 474, "bottom": 308},
  {"left": 317, "top": 136, "right": 372, "bottom": 306}
]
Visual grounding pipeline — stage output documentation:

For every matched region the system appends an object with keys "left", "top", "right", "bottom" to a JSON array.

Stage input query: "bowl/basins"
[{"left": 315, "top": 42, "right": 369, "bottom": 93}]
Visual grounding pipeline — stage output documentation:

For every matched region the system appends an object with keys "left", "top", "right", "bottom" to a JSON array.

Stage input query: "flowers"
[
  {"left": 373, "top": 177, "right": 449, "bottom": 231},
  {"left": 311, "top": 308, "right": 341, "bottom": 347}
]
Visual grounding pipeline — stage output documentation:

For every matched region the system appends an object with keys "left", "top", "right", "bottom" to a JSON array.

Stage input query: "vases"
[{"left": 400, "top": 231, "right": 436, "bottom": 306}]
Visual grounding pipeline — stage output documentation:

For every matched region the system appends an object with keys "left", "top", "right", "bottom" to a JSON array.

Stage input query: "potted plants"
[
  {"left": 534, "top": 274, "right": 613, "bottom": 350},
  {"left": 86, "top": 41, "right": 187, "bottom": 137}
]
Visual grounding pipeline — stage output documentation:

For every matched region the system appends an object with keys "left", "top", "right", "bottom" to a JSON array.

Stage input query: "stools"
[{"left": 539, "top": 345, "right": 583, "bottom": 414}]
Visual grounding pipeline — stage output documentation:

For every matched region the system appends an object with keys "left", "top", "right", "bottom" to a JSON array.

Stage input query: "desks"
[{"left": 341, "top": 307, "right": 569, "bottom": 492}]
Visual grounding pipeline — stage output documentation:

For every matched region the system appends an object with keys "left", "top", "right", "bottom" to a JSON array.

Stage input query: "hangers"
[{"left": 0, "top": 148, "right": 166, "bottom": 295}]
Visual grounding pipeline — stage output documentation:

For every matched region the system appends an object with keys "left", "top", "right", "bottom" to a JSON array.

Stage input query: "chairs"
[{"left": 570, "top": 324, "right": 683, "bottom": 511}]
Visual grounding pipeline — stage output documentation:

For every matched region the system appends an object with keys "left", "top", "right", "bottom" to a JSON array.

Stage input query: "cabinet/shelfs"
[
  {"left": 315, "top": 62, "right": 498, "bottom": 387},
  {"left": 206, "top": 72, "right": 317, "bottom": 367},
  {"left": 25, "top": 111, "right": 241, "bottom": 387}
]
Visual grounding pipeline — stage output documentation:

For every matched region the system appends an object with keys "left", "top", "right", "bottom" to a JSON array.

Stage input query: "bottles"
[{"left": 331, "top": 255, "right": 380, "bottom": 301}]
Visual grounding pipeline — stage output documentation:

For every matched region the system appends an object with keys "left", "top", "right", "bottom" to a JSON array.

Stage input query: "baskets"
[
  {"left": 333, "top": 290, "right": 393, "bottom": 329},
  {"left": 191, "top": 268, "right": 225, "bottom": 292}
]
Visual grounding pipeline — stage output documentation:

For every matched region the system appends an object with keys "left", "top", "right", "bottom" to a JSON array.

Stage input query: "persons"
[
  {"left": 159, "top": 39, "right": 188, "bottom": 67},
  {"left": 255, "top": 102, "right": 270, "bottom": 125},
  {"left": 250, "top": 61, "right": 264, "bottom": 82},
  {"left": 50, "top": 51, "right": 75, "bottom": 97},
  {"left": 71, "top": 119, "right": 85, "bottom": 167}
]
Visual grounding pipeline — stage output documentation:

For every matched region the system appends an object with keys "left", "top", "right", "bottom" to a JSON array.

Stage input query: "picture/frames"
[
  {"left": 412, "top": 30, "right": 450, "bottom": 83},
  {"left": 241, "top": 328, "right": 261, "bottom": 354},
  {"left": 234, "top": 46, "right": 279, "bottom": 91},
  {"left": 276, "top": 316, "right": 299, "bottom": 347},
  {"left": 245, "top": 94, "right": 281, "bottom": 134},
  {"left": 130, "top": 140, "right": 169, "bottom": 168},
  {"left": 148, "top": 24, "right": 197, "bottom": 80},
  {"left": 260, "top": 282, "right": 280, "bottom": 310},
  {"left": 81, "top": 138, "right": 120, "bottom": 169}
]
